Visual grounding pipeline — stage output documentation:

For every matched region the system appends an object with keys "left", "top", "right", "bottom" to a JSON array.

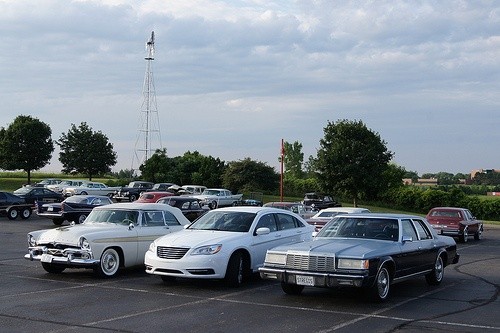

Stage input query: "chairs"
[
  {"left": 228, "top": 215, "right": 242, "bottom": 224},
  {"left": 250, "top": 216, "right": 271, "bottom": 229}
]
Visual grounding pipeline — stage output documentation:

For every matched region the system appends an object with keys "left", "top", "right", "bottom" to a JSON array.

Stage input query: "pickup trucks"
[{"left": 189, "top": 189, "right": 244, "bottom": 210}]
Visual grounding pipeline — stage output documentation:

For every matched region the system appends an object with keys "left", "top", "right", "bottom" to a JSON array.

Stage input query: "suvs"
[
  {"left": 303, "top": 193, "right": 342, "bottom": 210},
  {"left": 168, "top": 184, "right": 208, "bottom": 196}
]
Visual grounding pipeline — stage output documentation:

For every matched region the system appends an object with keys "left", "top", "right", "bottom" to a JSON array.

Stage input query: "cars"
[
  {"left": 43, "top": 179, "right": 92, "bottom": 193},
  {"left": 62, "top": 182, "right": 119, "bottom": 199},
  {"left": 262, "top": 202, "right": 319, "bottom": 219},
  {"left": 24, "top": 202, "right": 192, "bottom": 278},
  {"left": 112, "top": 181, "right": 155, "bottom": 202},
  {"left": 143, "top": 206, "right": 316, "bottom": 287},
  {"left": 0, "top": 192, "right": 37, "bottom": 220},
  {"left": 13, "top": 187, "right": 65, "bottom": 207},
  {"left": 131, "top": 192, "right": 174, "bottom": 203},
  {"left": 306, "top": 207, "right": 371, "bottom": 232},
  {"left": 425, "top": 207, "right": 484, "bottom": 243},
  {"left": 155, "top": 196, "right": 205, "bottom": 222},
  {"left": 146, "top": 183, "right": 173, "bottom": 192},
  {"left": 258, "top": 213, "right": 460, "bottom": 302},
  {"left": 233, "top": 198, "right": 263, "bottom": 207},
  {"left": 22, "top": 179, "right": 63, "bottom": 188},
  {"left": 36, "top": 193, "right": 113, "bottom": 225}
]
